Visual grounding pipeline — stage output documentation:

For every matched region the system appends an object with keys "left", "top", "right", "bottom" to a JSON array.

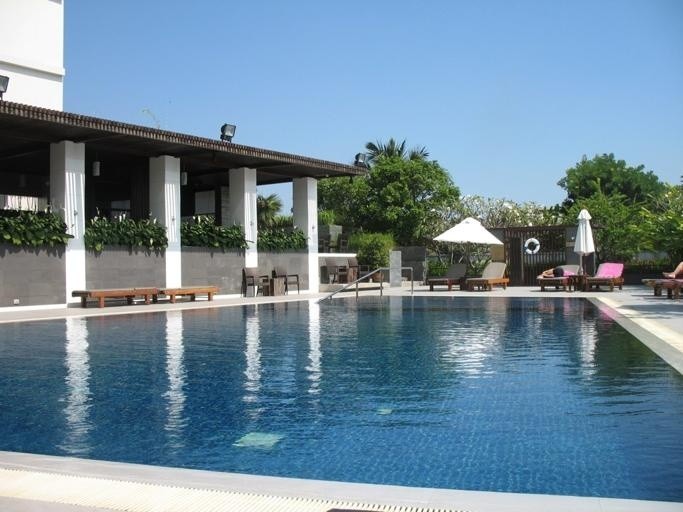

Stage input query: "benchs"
[
  {"left": 72, "top": 286, "right": 159, "bottom": 308},
  {"left": 157, "top": 286, "right": 220, "bottom": 303}
]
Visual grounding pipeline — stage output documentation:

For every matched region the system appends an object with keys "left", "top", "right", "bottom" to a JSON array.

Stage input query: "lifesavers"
[{"left": 524, "top": 238, "right": 541, "bottom": 255}]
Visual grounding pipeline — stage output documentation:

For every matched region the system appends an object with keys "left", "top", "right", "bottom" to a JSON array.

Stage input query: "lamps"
[
  {"left": 92, "top": 150, "right": 101, "bottom": 177},
  {"left": 354, "top": 152, "right": 366, "bottom": 167},
  {"left": 0, "top": 75, "right": 10, "bottom": 99},
  {"left": 181, "top": 164, "right": 188, "bottom": 185},
  {"left": 348, "top": 176, "right": 353, "bottom": 186},
  {"left": 220, "top": 123, "right": 236, "bottom": 143}
]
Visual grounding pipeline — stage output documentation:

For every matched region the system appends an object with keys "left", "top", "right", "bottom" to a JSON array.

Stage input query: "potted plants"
[{"left": 355, "top": 237, "right": 390, "bottom": 282}]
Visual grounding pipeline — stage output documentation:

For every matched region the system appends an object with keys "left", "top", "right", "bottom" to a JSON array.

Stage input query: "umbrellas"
[
  {"left": 573, "top": 209, "right": 596, "bottom": 273},
  {"left": 432, "top": 216, "right": 505, "bottom": 276}
]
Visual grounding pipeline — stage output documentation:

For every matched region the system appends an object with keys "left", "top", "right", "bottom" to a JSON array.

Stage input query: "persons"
[{"left": 662, "top": 262, "right": 683, "bottom": 278}]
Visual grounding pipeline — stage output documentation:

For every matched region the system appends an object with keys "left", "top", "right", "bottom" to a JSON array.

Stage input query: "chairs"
[
  {"left": 272, "top": 265, "right": 300, "bottom": 294},
  {"left": 641, "top": 278, "right": 683, "bottom": 299},
  {"left": 585, "top": 263, "right": 625, "bottom": 292},
  {"left": 347, "top": 256, "right": 375, "bottom": 282},
  {"left": 324, "top": 257, "right": 347, "bottom": 284},
  {"left": 467, "top": 261, "right": 510, "bottom": 292},
  {"left": 240, "top": 267, "right": 270, "bottom": 297},
  {"left": 427, "top": 263, "right": 474, "bottom": 290},
  {"left": 536, "top": 264, "right": 585, "bottom": 291}
]
